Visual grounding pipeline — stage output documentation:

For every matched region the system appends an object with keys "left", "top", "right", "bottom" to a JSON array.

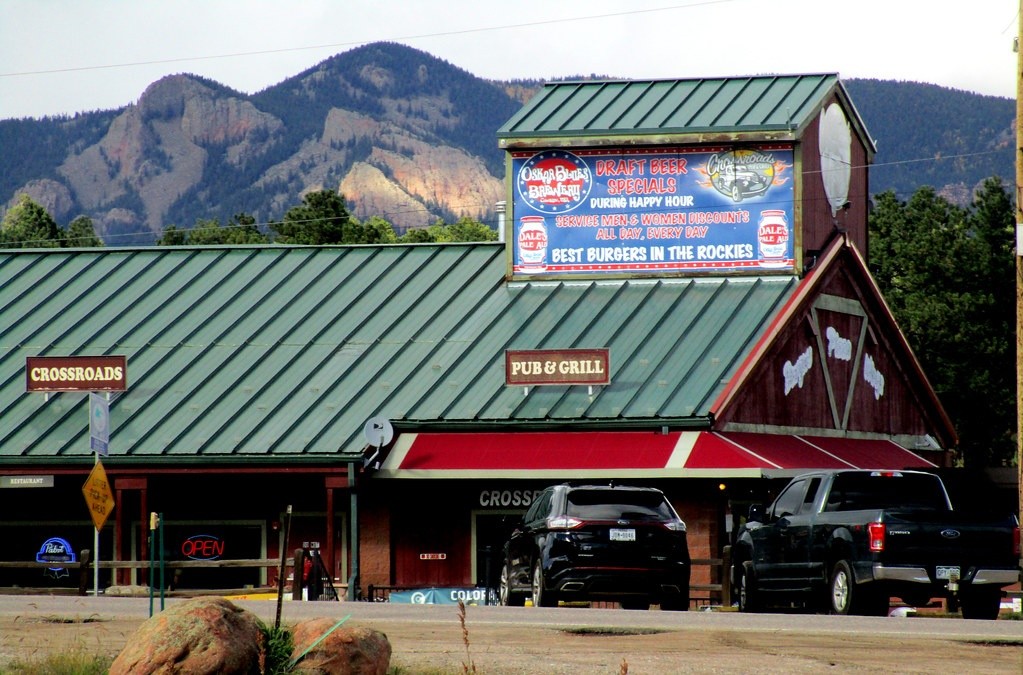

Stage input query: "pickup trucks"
[{"left": 730, "top": 470, "right": 1019, "bottom": 620}]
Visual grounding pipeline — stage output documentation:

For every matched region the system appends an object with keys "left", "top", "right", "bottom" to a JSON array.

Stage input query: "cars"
[{"left": 499, "top": 482, "right": 689, "bottom": 611}]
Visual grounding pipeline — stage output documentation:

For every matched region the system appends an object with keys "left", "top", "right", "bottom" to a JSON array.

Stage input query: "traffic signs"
[{"left": 25, "top": 355, "right": 127, "bottom": 392}]
